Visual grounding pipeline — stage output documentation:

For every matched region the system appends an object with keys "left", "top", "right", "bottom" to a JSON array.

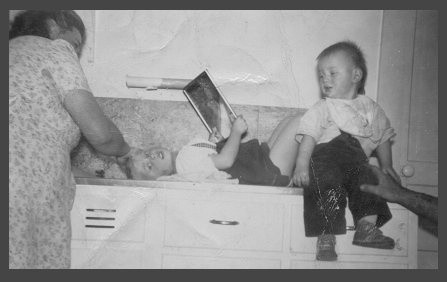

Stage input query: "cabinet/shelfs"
[{"left": 70, "top": 176, "right": 418, "bottom": 269}]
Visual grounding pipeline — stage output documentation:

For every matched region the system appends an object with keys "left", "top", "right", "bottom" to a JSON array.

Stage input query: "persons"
[
  {"left": 8, "top": 8, "right": 128, "bottom": 269},
  {"left": 288, "top": 39, "right": 403, "bottom": 260},
  {"left": 124, "top": 113, "right": 303, "bottom": 190},
  {"left": 360, "top": 161, "right": 438, "bottom": 226}
]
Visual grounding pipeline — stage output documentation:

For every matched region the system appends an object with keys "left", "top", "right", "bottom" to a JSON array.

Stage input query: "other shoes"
[
  {"left": 317, "top": 233, "right": 338, "bottom": 261},
  {"left": 352, "top": 223, "right": 395, "bottom": 249}
]
